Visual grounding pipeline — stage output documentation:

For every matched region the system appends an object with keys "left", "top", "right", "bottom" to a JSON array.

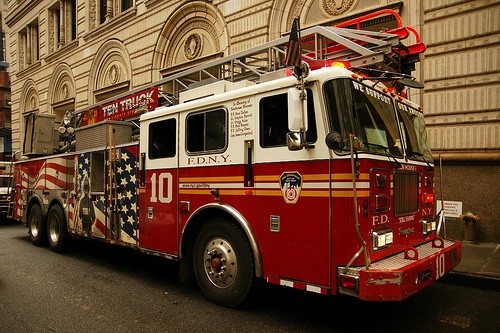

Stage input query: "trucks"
[{"left": 0, "top": 162, "right": 16, "bottom": 221}]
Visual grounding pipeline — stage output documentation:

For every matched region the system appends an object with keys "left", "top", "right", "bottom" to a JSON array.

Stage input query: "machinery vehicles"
[{"left": 7, "top": 10, "right": 463, "bottom": 309}]
